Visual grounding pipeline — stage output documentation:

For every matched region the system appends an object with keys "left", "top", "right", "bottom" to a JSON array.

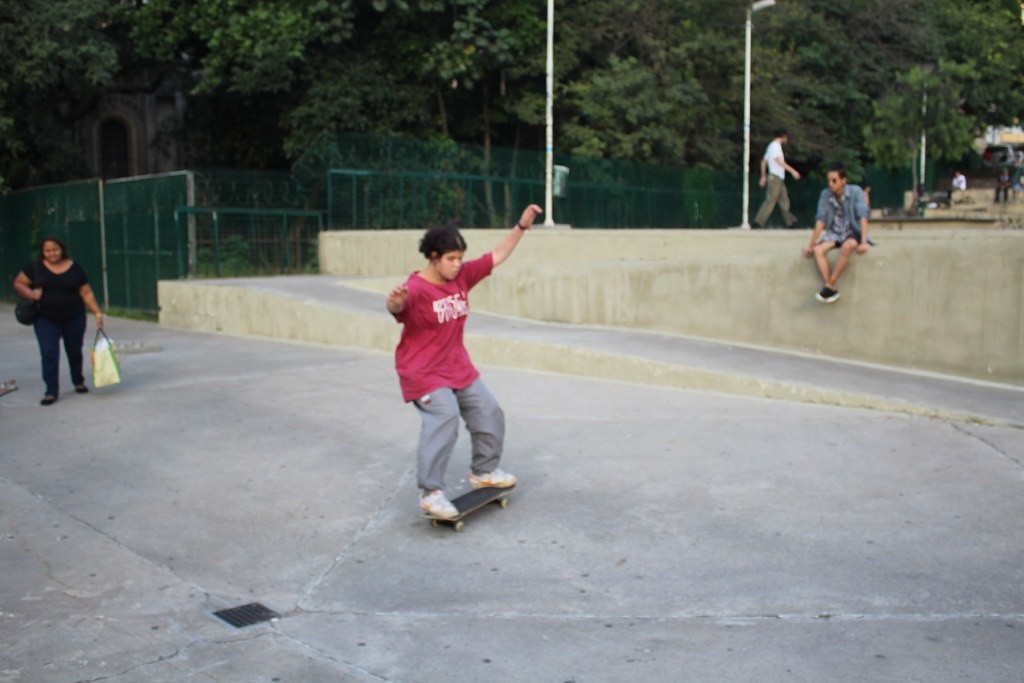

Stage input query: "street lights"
[{"left": 740, "top": 0, "right": 778, "bottom": 232}]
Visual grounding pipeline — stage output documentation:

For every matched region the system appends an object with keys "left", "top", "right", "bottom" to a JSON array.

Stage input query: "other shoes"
[
  {"left": 40, "top": 396, "right": 55, "bottom": 405},
  {"left": 75, "top": 387, "right": 88, "bottom": 393},
  {"left": 816, "top": 287, "right": 840, "bottom": 303}
]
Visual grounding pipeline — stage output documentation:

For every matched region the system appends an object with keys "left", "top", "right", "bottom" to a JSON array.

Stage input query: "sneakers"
[
  {"left": 418, "top": 489, "right": 459, "bottom": 518},
  {"left": 468, "top": 468, "right": 516, "bottom": 489}
]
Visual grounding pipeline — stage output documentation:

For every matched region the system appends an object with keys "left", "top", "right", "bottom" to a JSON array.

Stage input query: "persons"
[
  {"left": 995, "top": 165, "right": 1012, "bottom": 202},
  {"left": 805, "top": 161, "right": 869, "bottom": 303},
  {"left": 386, "top": 204, "right": 542, "bottom": 519},
  {"left": 948, "top": 171, "right": 967, "bottom": 200},
  {"left": 13, "top": 237, "right": 104, "bottom": 404},
  {"left": 751, "top": 128, "right": 807, "bottom": 228}
]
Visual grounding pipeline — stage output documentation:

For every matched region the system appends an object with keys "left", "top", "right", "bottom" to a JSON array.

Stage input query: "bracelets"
[
  {"left": 96, "top": 313, "right": 101, "bottom": 317},
  {"left": 518, "top": 222, "right": 526, "bottom": 230},
  {"left": 861, "top": 240, "right": 866, "bottom": 243}
]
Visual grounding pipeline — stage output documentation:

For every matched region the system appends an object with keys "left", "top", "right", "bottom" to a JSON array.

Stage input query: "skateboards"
[{"left": 418, "top": 482, "right": 516, "bottom": 532}]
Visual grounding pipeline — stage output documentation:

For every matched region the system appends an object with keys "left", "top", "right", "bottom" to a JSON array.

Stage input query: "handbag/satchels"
[
  {"left": 89, "top": 328, "right": 121, "bottom": 388},
  {"left": 14, "top": 299, "right": 39, "bottom": 325}
]
